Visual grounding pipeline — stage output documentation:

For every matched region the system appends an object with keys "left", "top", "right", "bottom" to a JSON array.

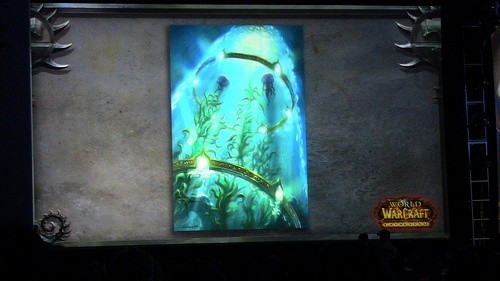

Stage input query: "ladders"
[{"left": 459, "top": 15, "right": 495, "bottom": 252}]
